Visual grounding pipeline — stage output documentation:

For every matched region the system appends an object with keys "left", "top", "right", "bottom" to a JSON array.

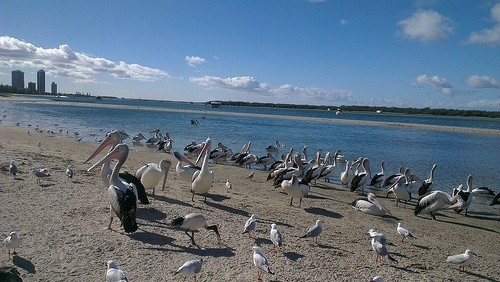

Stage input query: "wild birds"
[
  {"left": 367, "top": 229, "right": 398, "bottom": 264},
  {"left": 66, "top": 165, "right": 73, "bottom": 178},
  {"left": 87, "top": 143, "right": 139, "bottom": 233},
  {"left": 173, "top": 259, "right": 203, "bottom": 282},
  {"left": 31, "top": 169, "right": 52, "bottom": 184},
  {"left": 299, "top": 220, "right": 324, "bottom": 244},
  {"left": 105, "top": 260, "right": 128, "bottom": 282},
  {"left": 173, "top": 137, "right": 344, "bottom": 208},
  {"left": 270, "top": 223, "right": 283, "bottom": 249},
  {"left": 36, "top": 141, "right": 42, "bottom": 148},
  {"left": 172, "top": 213, "right": 221, "bottom": 250},
  {"left": 445, "top": 249, "right": 476, "bottom": 272},
  {"left": 341, "top": 157, "right": 500, "bottom": 221},
  {"left": 132, "top": 128, "right": 175, "bottom": 154},
  {"left": 396, "top": 223, "right": 417, "bottom": 242},
  {"left": 83, "top": 130, "right": 150, "bottom": 205},
  {"left": 9, "top": 160, "right": 18, "bottom": 176},
  {"left": 252, "top": 246, "right": 274, "bottom": 280},
  {"left": 135, "top": 158, "right": 173, "bottom": 195},
  {"left": 2, "top": 232, "right": 21, "bottom": 259},
  {"left": 370, "top": 276, "right": 384, "bottom": 282},
  {"left": 242, "top": 214, "right": 259, "bottom": 238}
]
